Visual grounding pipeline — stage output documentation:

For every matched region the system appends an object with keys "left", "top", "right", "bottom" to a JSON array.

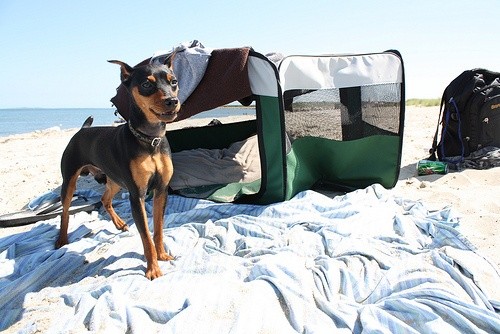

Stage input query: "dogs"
[{"left": 54, "top": 48, "right": 186, "bottom": 282}]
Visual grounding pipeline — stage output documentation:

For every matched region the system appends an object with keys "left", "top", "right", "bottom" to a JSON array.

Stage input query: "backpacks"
[{"left": 431, "top": 69, "right": 500, "bottom": 172}]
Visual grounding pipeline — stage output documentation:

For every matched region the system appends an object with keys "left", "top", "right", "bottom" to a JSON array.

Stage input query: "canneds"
[{"left": 415, "top": 160, "right": 450, "bottom": 175}]
